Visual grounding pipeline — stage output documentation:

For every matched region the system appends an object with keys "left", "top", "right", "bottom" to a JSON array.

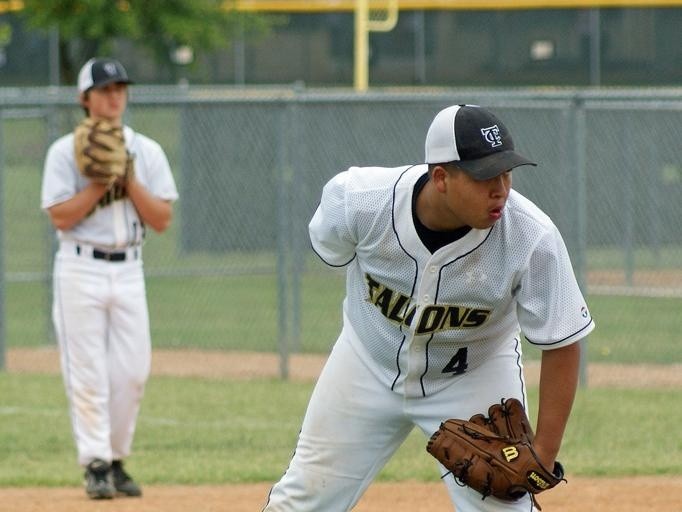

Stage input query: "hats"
[
  {"left": 425, "top": 103, "right": 537, "bottom": 182},
  {"left": 77, "top": 57, "right": 135, "bottom": 93}
]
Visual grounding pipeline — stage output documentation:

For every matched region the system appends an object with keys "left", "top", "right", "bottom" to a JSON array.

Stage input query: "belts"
[{"left": 75, "top": 244, "right": 128, "bottom": 262}]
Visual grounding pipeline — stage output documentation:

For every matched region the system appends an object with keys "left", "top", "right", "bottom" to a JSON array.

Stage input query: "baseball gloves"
[
  {"left": 72, "top": 114, "right": 129, "bottom": 186},
  {"left": 426, "top": 397, "right": 566, "bottom": 503}
]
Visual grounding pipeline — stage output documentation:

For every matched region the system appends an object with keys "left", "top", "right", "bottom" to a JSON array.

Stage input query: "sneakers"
[{"left": 84, "top": 460, "right": 143, "bottom": 497}]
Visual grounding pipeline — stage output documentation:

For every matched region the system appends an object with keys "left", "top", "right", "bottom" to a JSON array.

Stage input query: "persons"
[
  {"left": 257, "top": 102, "right": 597, "bottom": 511},
  {"left": 39, "top": 56, "right": 181, "bottom": 503}
]
